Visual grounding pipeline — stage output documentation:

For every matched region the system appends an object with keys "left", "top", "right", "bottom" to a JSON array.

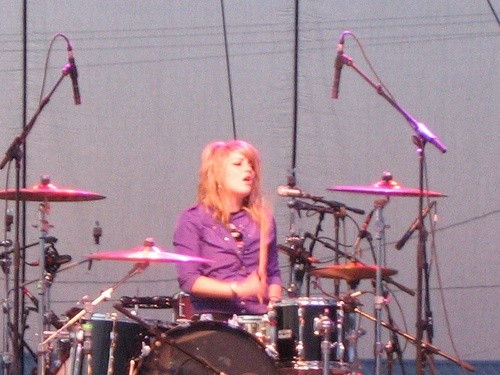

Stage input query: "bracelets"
[{"left": 231, "top": 282, "right": 238, "bottom": 301}]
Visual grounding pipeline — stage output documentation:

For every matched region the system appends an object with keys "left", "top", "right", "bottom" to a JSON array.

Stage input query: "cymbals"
[
  {"left": 323, "top": 179, "right": 448, "bottom": 197},
  {"left": 305, "top": 261, "right": 399, "bottom": 281},
  {"left": 82, "top": 244, "right": 217, "bottom": 264},
  {"left": 0, "top": 182, "right": 108, "bottom": 202}
]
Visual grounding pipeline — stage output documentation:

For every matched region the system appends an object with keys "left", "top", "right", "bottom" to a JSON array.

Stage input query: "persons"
[{"left": 171, "top": 139, "right": 282, "bottom": 322}]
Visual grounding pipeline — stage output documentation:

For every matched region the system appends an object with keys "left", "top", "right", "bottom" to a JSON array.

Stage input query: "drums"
[
  {"left": 231, "top": 313, "right": 278, "bottom": 348},
  {"left": 69, "top": 314, "right": 178, "bottom": 375},
  {"left": 133, "top": 319, "right": 279, "bottom": 375},
  {"left": 271, "top": 296, "right": 360, "bottom": 375}
]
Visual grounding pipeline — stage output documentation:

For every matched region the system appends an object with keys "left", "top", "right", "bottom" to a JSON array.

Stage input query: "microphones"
[
  {"left": 276, "top": 243, "right": 320, "bottom": 264},
  {"left": 21, "top": 288, "right": 63, "bottom": 330},
  {"left": 67, "top": 45, "right": 81, "bottom": 105},
  {"left": 286, "top": 200, "right": 335, "bottom": 214},
  {"left": 277, "top": 184, "right": 310, "bottom": 198},
  {"left": 394, "top": 203, "right": 434, "bottom": 250},
  {"left": 331, "top": 38, "right": 345, "bottom": 98}
]
